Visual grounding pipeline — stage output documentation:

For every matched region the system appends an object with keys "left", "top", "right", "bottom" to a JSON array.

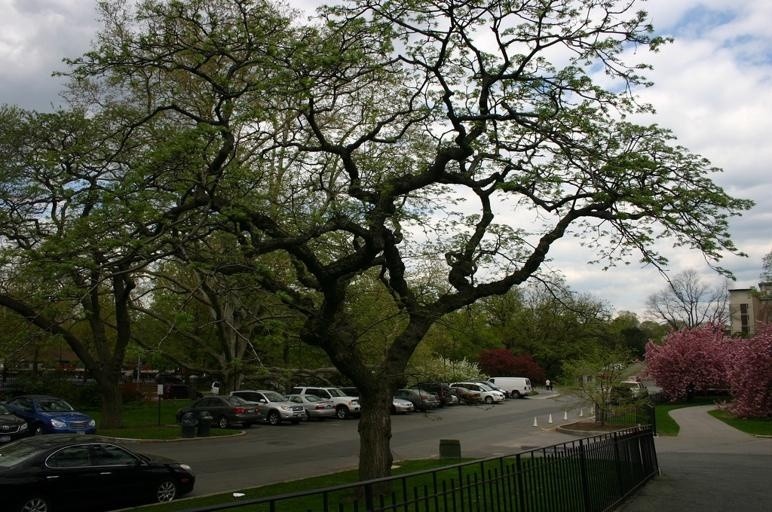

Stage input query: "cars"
[
  {"left": 391, "top": 394, "right": 415, "bottom": 415},
  {"left": 0, "top": 433, "right": 195, "bottom": 512},
  {"left": 283, "top": 393, "right": 338, "bottom": 423},
  {"left": 175, "top": 394, "right": 262, "bottom": 431},
  {"left": 1, "top": 394, "right": 97, "bottom": 435},
  {"left": 396, "top": 380, "right": 509, "bottom": 410},
  {"left": 1, "top": 405, "right": 31, "bottom": 447}
]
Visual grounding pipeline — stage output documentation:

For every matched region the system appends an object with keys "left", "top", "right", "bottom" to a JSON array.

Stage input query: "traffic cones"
[{"left": 530, "top": 405, "right": 595, "bottom": 428}]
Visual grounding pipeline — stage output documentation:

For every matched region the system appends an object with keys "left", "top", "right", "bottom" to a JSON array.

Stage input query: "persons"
[
  {"left": 545, "top": 378, "right": 553, "bottom": 390},
  {"left": 635, "top": 376, "right": 642, "bottom": 382}
]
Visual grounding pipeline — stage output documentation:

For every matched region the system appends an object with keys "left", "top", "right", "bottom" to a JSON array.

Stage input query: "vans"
[
  {"left": 228, "top": 388, "right": 306, "bottom": 427},
  {"left": 620, "top": 380, "right": 649, "bottom": 400},
  {"left": 291, "top": 385, "right": 362, "bottom": 421},
  {"left": 607, "top": 386, "right": 633, "bottom": 405},
  {"left": 487, "top": 376, "right": 532, "bottom": 399}
]
